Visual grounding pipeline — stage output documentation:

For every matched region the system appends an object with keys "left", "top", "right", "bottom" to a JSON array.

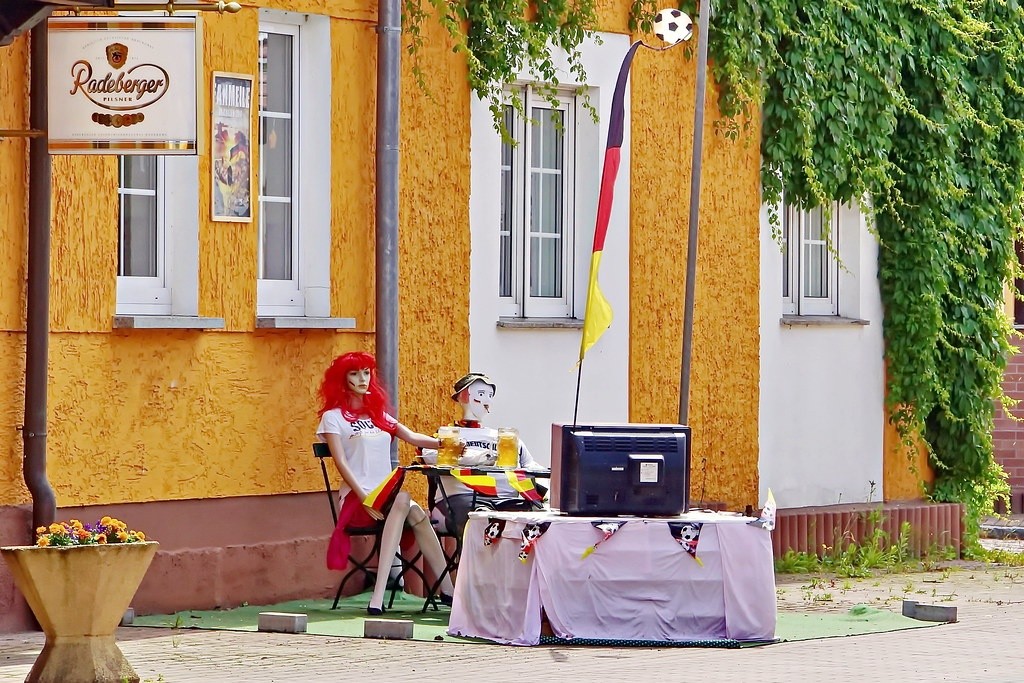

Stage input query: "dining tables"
[{"left": 401, "top": 462, "right": 552, "bottom": 612}]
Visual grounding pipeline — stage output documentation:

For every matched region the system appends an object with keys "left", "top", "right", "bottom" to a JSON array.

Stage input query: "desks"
[{"left": 448, "top": 510, "right": 778, "bottom": 647}]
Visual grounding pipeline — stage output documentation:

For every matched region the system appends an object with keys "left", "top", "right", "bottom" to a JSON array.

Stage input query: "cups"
[
  {"left": 497, "top": 427, "right": 519, "bottom": 468},
  {"left": 436, "top": 426, "right": 463, "bottom": 467}
]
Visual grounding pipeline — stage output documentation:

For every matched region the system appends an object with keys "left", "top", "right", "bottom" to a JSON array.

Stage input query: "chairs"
[
  {"left": 313, "top": 440, "right": 455, "bottom": 612},
  {"left": 416, "top": 444, "right": 460, "bottom": 572}
]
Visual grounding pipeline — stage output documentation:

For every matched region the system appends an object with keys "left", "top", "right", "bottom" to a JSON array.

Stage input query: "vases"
[{"left": 0, "top": 542, "right": 165, "bottom": 683}]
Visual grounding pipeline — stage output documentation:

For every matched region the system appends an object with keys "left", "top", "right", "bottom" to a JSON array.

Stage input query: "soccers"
[{"left": 651, "top": 6, "right": 694, "bottom": 44}]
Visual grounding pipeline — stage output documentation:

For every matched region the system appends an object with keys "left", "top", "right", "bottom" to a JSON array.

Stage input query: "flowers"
[{"left": 34, "top": 515, "right": 145, "bottom": 546}]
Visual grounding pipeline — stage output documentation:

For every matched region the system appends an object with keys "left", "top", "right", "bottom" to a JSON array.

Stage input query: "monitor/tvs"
[{"left": 551, "top": 423, "right": 691, "bottom": 517}]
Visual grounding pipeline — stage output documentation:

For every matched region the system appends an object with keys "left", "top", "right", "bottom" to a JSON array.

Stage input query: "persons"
[
  {"left": 417, "top": 373, "right": 549, "bottom": 540},
  {"left": 316, "top": 351, "right": 468, "bottom": 615}
]
